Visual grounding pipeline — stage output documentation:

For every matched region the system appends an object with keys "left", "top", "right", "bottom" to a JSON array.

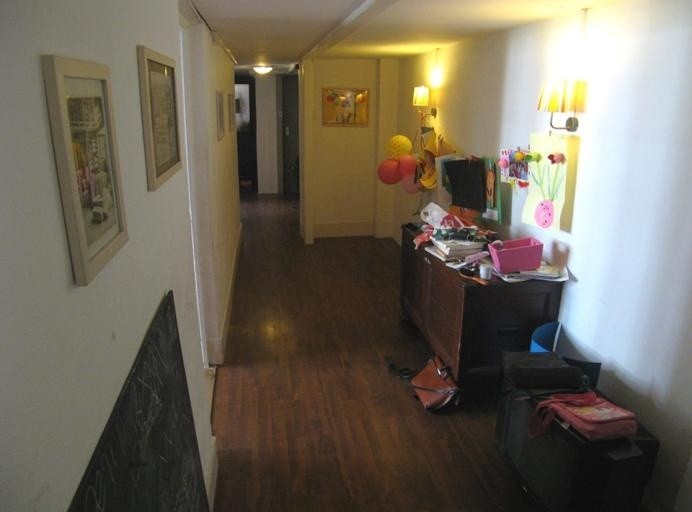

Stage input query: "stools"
[{"left": 500, "top": 383, "right": 660, "bottom": 512}]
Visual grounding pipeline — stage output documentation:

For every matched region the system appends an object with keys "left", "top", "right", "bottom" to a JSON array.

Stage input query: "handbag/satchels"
[
  {"left": 420, "top": 202, "right": 462, "bottom": 228},
  {"left": 503, "top": 352, "right": 580, "bottom": 388},
  {"left": 410, "top": 355, "right": 459, "bottom": 411},
  {"left": 549, "top": 390, "right": 638, "bottom": 440}
]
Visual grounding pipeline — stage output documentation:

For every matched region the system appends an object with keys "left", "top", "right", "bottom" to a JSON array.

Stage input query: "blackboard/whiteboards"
[{"left": 67, "top": 290, "right": 209, "bottom": 512}]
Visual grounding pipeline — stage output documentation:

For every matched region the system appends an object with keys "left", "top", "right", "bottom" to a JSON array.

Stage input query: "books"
[{"left": 425, "top": 235, "right": 486, "bottom": 263}]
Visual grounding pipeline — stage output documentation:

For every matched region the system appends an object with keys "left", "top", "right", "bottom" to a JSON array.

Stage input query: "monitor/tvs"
[{"left": 442, "top": 161, "right": 487, "bottom": 212}]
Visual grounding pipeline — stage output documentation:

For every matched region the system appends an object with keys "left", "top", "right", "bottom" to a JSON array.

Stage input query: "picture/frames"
[
  {"left": 136, "top": 44, "right": 184, "bottom": 192},
  {"left": 321, "top": 86, "right": 369, "bottom": 127},
  {"left": 41, "top": 54, "right": 129, "bottom": 287}
]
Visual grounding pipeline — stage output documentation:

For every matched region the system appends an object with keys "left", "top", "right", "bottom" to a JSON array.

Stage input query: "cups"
[{"left": 479, "top": 264, "right": 492, "bottom": 280}]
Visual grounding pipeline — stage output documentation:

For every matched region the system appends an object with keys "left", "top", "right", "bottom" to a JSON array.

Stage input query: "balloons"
[{"left": 378, "top": 134, "right": 424, "bottom": 193}]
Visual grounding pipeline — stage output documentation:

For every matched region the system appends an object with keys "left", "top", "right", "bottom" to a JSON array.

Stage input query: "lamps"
[
  {"left": 412, "top": 86, "right": 437, "bottom": 118},
  {"left": 537, "top": 77, "right": 587, "bottom": 132},
  {"left": 253, "top": 65, "right": 273, "bottom": 75}
]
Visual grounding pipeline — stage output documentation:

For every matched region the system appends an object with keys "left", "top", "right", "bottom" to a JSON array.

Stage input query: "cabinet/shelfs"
[{"left": 401, "top": 222, "right": 563, "bottom": 384}]
